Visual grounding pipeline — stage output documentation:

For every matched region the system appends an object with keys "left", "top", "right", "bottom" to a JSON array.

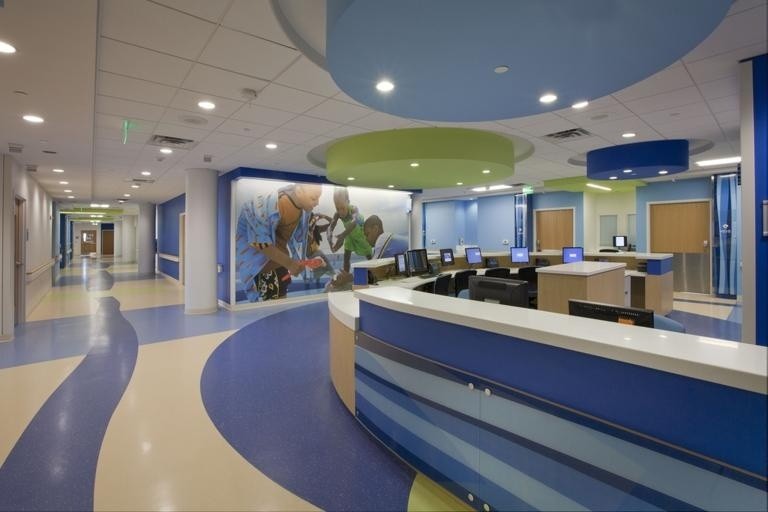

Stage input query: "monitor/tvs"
[
  {"left": 612, "top": 235, "right": 627, "bottom": 247},
  {"left": 567, "top": 299, "right": 654, "bottom": 329},
  {"left": 407, "top": 249, "right": 429, "bottom": 276},
  {"left": 465, "top": 247, "right": 483, "bottom": 265},
  {"left": 562, "top": 247, "right": 584, "bottom": 264},
  {"left": 510, "top": 247, "right": 530, "bottom": 264}
]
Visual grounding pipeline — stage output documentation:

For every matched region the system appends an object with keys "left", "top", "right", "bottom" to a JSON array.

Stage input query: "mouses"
[{"left": 439, "top": 274, "right": 442, "bottom": 276}]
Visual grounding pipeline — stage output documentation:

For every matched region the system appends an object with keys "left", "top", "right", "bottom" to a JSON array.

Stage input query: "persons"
[{"left": 236, "top": 179, "right": 410, "bottom": 302}]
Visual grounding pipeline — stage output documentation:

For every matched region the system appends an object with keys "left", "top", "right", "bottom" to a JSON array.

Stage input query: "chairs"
[
  {"left": 454, "top": 270, "right": 476, "bottom": 297},
  {"left": 518, "top": 265, "right": 538, "bottom": 308},
  {"left": 652, "top": 313, "right": 687, "bottom": 333},
  {"left": 485, "top": 268, "right": 510, "bottom": 278},
  {"left": 425, "top": 273, "right": 452, "bottom": 296}
]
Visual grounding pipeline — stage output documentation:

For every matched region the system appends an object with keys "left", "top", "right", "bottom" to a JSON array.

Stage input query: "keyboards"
[{"left": 422, "top": 272, "right": 437, "bottom": 279}]
